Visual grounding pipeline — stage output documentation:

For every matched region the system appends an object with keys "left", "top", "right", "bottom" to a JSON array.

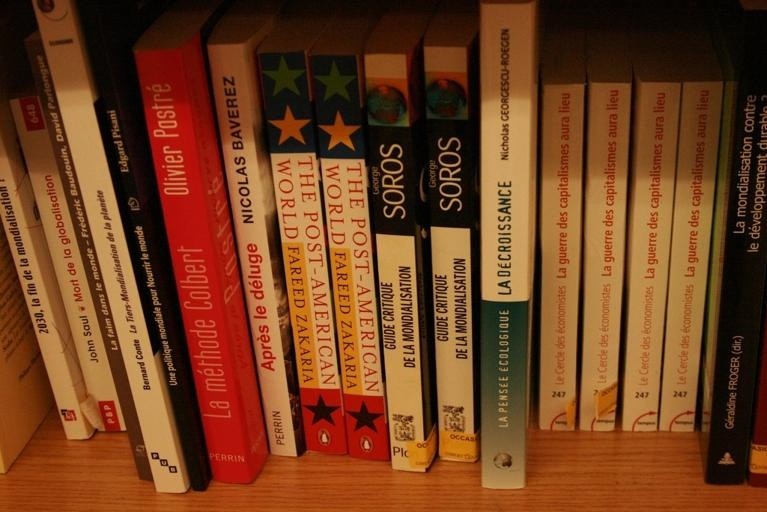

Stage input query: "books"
[{"left": 1, "top": 1, "right": 766, "bottom": 495}]
